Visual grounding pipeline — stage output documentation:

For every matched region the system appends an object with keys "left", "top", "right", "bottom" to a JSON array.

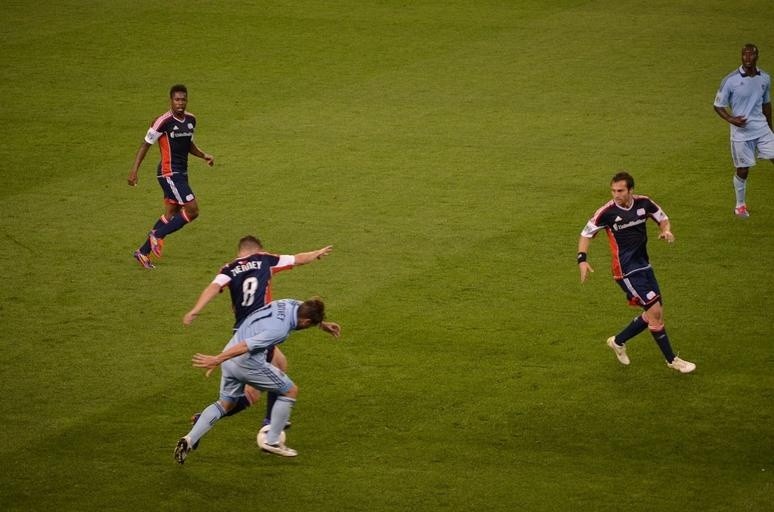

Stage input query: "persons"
[
  {"left": 714, "top": 44, "right": 774, "bottom": 217},
  {"left": 577, "top": 171, "right": 696, "bottom": 375},
  {"left": 173, "top": 297, "right": 340, "bottom": 465},
  {"left": 182, "top": 236, "right": 333, "bottom": 428},
  {"left": 127, "top": 86, "right": 214, "bottom": 269}
]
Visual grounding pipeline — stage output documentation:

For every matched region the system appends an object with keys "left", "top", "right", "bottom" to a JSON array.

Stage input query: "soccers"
[{"left": 257, "top": 425, "right": 286, "bottom": 447}]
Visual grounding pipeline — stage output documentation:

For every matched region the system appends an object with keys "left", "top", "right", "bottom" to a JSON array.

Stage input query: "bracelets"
[{"left": 578, "top": 253, "right": 586, "bottom": 264}]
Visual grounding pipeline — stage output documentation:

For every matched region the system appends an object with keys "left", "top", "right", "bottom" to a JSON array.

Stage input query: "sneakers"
[
  {"left": 148, "top": 229, "right": 163, "bottom": 259},
  {"left": 189, "top": 413, "right": 203, "bottom": 451},
  {"left": 664, "top": 356, "right": 697, "bottom": 373},
  {"left": 172, "top": 434, "right": 192, "bottom": 466},
  {"left": 132, "top": 247, "right": 156, "bottom": 270},
  {"left": 261, "top": 418, "right": 293, "bottom": 430},
  {"left": 260, "top": 440, "right": 299, "bottom": 458},
  {"left": 605, "top": 334, "right": 632, "bottom": 366},
  {"left": 733, "top": 203, "right": 751, "bottom": 219}
]
